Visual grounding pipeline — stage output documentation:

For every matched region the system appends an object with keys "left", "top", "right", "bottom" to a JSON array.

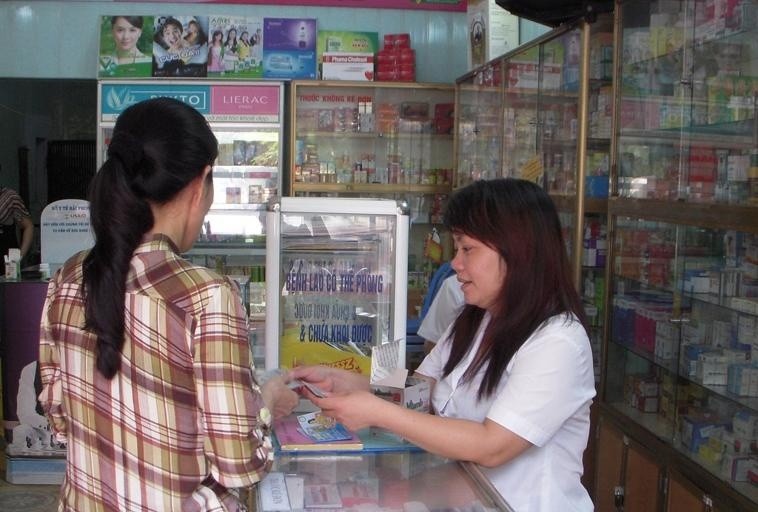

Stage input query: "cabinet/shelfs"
[
  {"left": 93, "top": 74, "right": 461, "bottom": 512},
  {"left": 456, "top": 1, "right": 758, "bottom": 512}
]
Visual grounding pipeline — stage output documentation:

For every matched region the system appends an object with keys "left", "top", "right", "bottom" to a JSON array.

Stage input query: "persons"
[
  {"left": 108, "top": 16, "right": 152, "bottom": 64},
  {"left": 415, "top": 274, "right": 464, "bottom": 355},
  {"left": 208, "top": 29, "right": 261, "bottom": 71},
  {"left": 161, "top": 18, "right": 203, "bottom": 58},
  {"left": 291, "top": 178, "right": 597, "bottom": 512},
  {"left": 39, "top": 97, "right": 298, "bottom": 512},
  {"left": 1, "top": 182, "right": 34, "bottom": 276}
]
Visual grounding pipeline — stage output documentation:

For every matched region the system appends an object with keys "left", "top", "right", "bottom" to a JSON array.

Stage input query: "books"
[{"left": 274, "top": 420, "right": 364, "bottom": 451}]
